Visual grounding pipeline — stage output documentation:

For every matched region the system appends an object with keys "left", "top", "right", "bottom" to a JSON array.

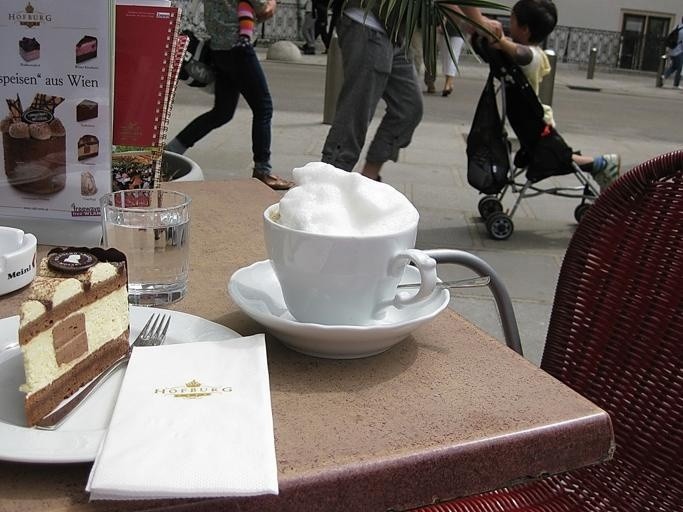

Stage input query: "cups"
[
  {"left": 261, "top": 200, "right": 438, "bottom": 324},
  {"left": 99, "top": 188, "right": 194, "bottom": 307}
]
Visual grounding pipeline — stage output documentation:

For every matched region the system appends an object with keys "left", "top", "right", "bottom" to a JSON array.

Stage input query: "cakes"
[
  {"left": 76, "top": 99, "right": 99, "bottom": 121},
  {"left": 77, "top": 135, "right": 99, "bottom": 161},
  {"left": 19, "top": 36, "right": 40, "bottom": 61},
  {"left": 17, "top": 242, "right": 134, "bottom": 428},
  {"left": 76, "top": 35, "right": 98, "bottom": 63}
]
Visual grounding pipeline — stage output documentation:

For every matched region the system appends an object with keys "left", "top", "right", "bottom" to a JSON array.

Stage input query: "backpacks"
[{"left": 668, "top": 24, "right": 683, "bottom": 48}]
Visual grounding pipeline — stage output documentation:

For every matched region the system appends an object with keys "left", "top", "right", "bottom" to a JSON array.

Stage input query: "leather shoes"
[{"left": 253, "top": 172, "right": 295, "bottom": 189}]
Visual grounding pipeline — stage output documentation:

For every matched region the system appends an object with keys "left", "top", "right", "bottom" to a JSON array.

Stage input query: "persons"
[
  {"left": 233, "top": 0, "right": 257, "bottom": 52},
  {"left": 489, "top": 0, "right": 621, "bottom": 190},
  {"left": 296, "top": 1, "right": 330, "bottom": 55},
  {"left": 316, "top": 0, "right": 507, "bottom": 183},
  {"left": 440, "top": 17, "right": 462, "bottom": 99},
  {"left": 657, "top": 16, "right": 683, "bottom": 90},
  {"left": 298, "top": 0, "right": 315, "bottom": 57},
  {"left": 408, "top": 13, "right": 448, "bottom": 95},
  {"left": 161, "top": 0, "right": 293, "bottom": 190}
]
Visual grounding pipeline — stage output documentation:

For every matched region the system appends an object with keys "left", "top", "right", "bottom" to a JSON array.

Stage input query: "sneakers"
[{"left": 592, "top": 153, "right": 620, "bottom": 194}]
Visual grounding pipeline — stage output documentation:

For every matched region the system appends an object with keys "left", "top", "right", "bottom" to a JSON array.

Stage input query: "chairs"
[{"left": 405, "top": 149, "right": 683, "bottom": 510}]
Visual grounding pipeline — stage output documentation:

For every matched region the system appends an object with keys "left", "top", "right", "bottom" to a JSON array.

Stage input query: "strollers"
[{"left": 466, "top": 31, "right": 605, "bottom": 241}]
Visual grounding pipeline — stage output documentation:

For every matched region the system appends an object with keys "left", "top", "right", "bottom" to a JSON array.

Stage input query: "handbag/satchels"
[{"left": 467, "top": 73, "right": 512, "bottom": 194}]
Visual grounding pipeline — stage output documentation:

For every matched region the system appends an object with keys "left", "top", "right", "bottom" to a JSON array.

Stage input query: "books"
[{"left": 2, "top": 1, "right": 189, "bottom": 249}]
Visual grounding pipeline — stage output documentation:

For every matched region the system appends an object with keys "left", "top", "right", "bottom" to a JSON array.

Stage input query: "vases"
[{"left": 159, "top": 150, "right": 204, "bottom": 182}]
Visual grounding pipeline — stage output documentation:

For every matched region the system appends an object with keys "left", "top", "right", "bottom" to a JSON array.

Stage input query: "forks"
[{"left": 31, "top": 311, "right": 175, "bottom": 430}]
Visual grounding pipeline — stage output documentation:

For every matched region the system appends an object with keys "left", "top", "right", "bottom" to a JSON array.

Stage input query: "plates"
[
  {"left": 0, "top": 304, "right": 240, "bottom": 464},
  {"left": 224, "top": 256, "right": 450, "bottom": 361}
]
